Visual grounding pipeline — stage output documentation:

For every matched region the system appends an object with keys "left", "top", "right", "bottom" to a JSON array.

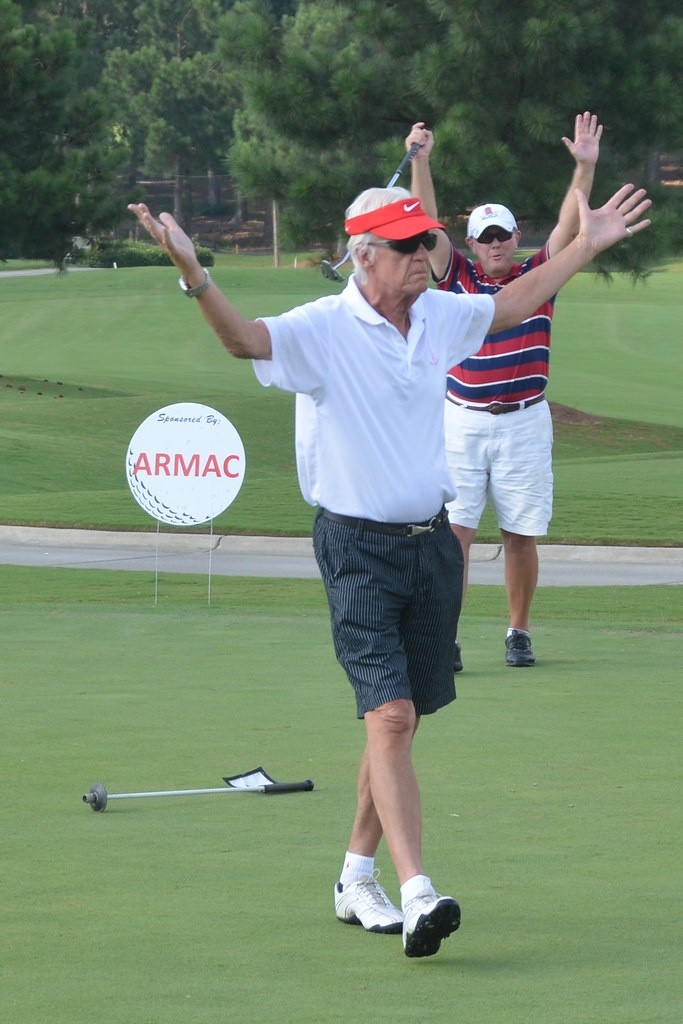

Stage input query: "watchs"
[{"left": 177, "top": 267, "right": 211, "bottom": 298}]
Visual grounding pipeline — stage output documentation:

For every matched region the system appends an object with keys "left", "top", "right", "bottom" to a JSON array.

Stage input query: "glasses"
[
  {"left": 369, "top": 233, "right": 437, "bottom": 254},
  {"left": 476, "top": 231, "right": 512, "bottom": 244}
]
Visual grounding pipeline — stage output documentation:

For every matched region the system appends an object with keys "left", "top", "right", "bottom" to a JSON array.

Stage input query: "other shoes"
[
  {"left": 504, "top": 630, "right": 535, "bottom": 667},
  {"left": 450, "top": 643, "right": 464, "bottom": 672}
]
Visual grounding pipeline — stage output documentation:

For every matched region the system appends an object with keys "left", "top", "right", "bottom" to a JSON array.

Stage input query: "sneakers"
[
  {"left": 334, "top": 868, "right": 403, "bottom": 934},
  {"left": 402, "top": 878, "right": 462, "bottom": 958}
]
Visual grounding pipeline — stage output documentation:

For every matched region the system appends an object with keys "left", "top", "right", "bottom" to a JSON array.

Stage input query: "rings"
[{"left": 626, "top": 227, "right": 634, "bottom": 237}]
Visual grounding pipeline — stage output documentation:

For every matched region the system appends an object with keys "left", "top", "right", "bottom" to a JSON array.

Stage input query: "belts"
[
  {"left": 446, "top": 393, "right": 545, "bottom": 415},
  {"left": 322, "top": 506, "right": 449, "bottom": 538}
]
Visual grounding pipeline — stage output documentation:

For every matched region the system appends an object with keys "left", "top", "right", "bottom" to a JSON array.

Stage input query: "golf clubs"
[{"left": 321, "top": 143, "right": 420, "bottom": 283}]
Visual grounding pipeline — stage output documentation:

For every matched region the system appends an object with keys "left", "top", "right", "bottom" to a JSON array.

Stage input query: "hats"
[
  {"left": 344, "top": 186, "right": 448, "bottom": 240},
  {"left": 467, "top": 203, "right": 518, "bottom": 239}
]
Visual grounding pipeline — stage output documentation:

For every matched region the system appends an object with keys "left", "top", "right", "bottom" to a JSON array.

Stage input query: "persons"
[
  {"left": 128, "top": 179, "right": 652, "bottom": 962},
  {"left": 406, "top": 110, "right": 604, "bottom": 674}
]
